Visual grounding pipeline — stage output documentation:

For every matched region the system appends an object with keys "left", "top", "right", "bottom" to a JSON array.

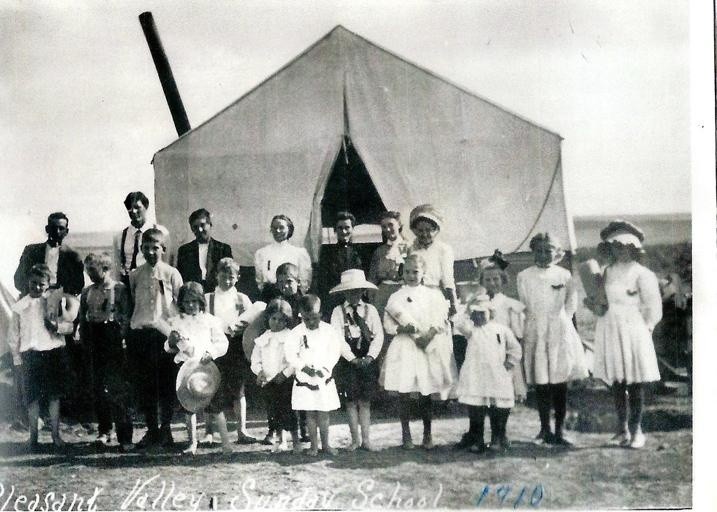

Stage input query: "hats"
[
  {"left": 175, "top": 356, "right": 222, "bottom": 413},
  {"left": 469, "top": 293, "right": 494, "bottom": 312},
  {"left": 408, "top": 204, "right": 444, "bottom": 236},
  {"left": 595, "top": 220, "right": 647, "bottom": 259},
  {"left": 328, "top": 268, "right": 379, "bottom": 294}
]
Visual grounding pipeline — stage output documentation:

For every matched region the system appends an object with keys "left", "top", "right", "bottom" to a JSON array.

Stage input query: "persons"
[
  {"left": 79, "top": 248, "right": 140, "bottom": 455},
  {"left": 7, "top": 263, "right": 79, "bottom": 455},
  {"left": 162, "top": 203, "right": 527, "bottom": 460},
  {"left": 583, "top": 219, "right": 665, "bottom": 450},
  {"left": 5, "top": 212, "right": 83, "bottom": 444},
  {"left": 516, "top": 230, "right": 591, "bottom": 452},
  {"left": 121, "top": 226, "right": 185, "bottom": 454},
  {"left": 108, "top": 191, "right": 172, "bottom": 292}
]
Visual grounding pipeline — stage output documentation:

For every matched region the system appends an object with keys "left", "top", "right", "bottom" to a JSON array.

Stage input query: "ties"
[{"left": 129, "top": 229, "right": 142, "bottom": 271}]
